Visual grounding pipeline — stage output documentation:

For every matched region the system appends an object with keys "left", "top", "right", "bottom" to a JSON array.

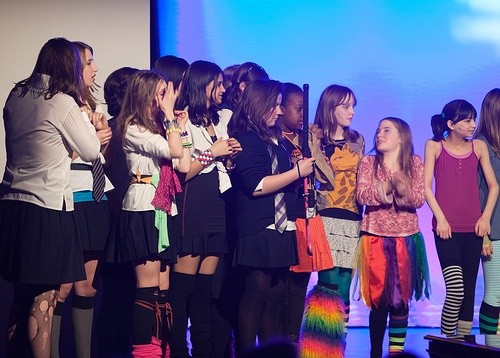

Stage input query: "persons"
[{"left": 0, "top": 35, "right": 500, "bottom": 358}]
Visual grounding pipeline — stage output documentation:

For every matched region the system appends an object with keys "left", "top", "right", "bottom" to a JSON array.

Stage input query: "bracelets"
[
  {"left": 198, "top": 149, "right": 214, "bottom": 167},
  {"left": 165, "top": 127, "right": 180, "bottom": 134},
  {"left": 164, "top": 118, "right": 178, "bottom": 127},
  {"left": 181, "top": 132, "right": 192, "bottom": 149}
]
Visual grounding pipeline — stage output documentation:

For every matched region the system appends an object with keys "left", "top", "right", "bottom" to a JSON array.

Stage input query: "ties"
[
  {"left": 92, "top": 158, "right": 105, "bottom": 203},
  {"left": 268, "top": 142, "right": 288, "bottom": 233}
]
